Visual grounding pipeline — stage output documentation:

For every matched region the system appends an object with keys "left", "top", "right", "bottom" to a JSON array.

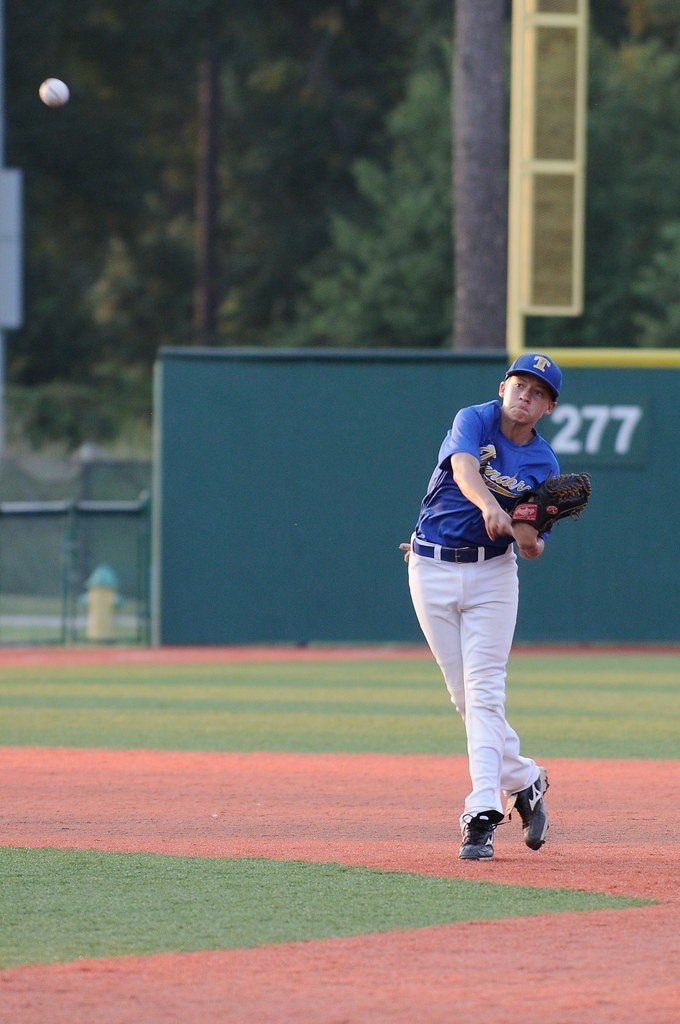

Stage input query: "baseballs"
[{"left": 39, "top": 77, "right": 71, "bottom": 108}]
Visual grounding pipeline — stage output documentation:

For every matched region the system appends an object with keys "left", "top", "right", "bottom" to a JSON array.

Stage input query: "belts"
[{"left": 413, "top": 540, "right": 507, "bottom": 563}]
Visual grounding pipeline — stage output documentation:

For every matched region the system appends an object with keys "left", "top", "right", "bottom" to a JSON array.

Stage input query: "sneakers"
[
  {"left": 458, "top": 809, "right": 504, "bottom": 861},
  {"left": 505, "top": 766, "right": 549, "bottom": 850}
]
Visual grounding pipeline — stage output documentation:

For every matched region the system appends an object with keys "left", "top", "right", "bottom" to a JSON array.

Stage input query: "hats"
[{"left": 506, "top": 353, "right": 563, "bottom": 398}]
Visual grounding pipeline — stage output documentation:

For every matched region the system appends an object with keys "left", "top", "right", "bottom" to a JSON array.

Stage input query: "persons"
[{"left": 407, "top": 353, "right": 565, "bottom": 862}]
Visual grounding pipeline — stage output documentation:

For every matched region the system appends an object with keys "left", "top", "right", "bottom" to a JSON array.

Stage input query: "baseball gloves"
[{"left": 510, "top": 473, "right": 592, "bottom": 533}]
